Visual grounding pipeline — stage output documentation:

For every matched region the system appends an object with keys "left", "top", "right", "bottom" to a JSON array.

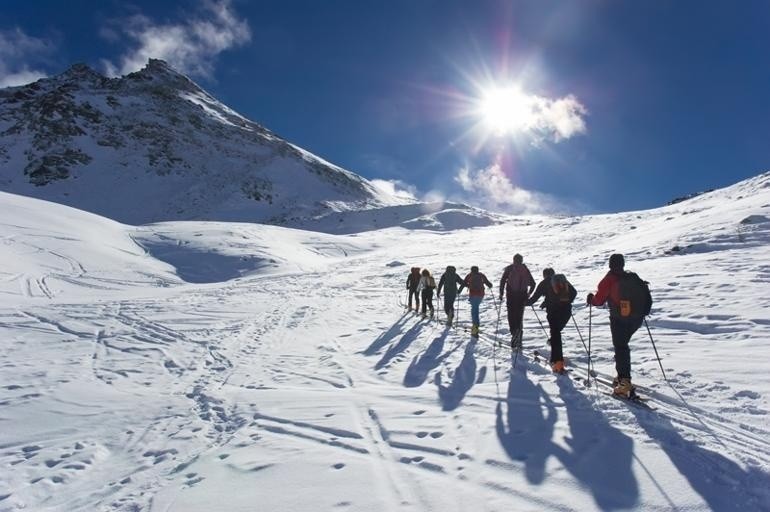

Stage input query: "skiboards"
[
  {"left": 398, "top": 301, "right": 419, "bottom": 313},
  {"left": 464, "top": 324, "right": 502, "bottom": 346},
  {"left": 416, "top": 312, "right": 439, "bottom": 322},
  {"left": 437, "top": 318, "right": 467, "bottom": 332},
  {"left": 499, "top": 340, "right": 541, "bottom": 363},
  {"left": 538, "top": 351, "right": 594, "bottom": 382},
  {"left": 583, "top": 370, "right": 660, "bottom": 410}
]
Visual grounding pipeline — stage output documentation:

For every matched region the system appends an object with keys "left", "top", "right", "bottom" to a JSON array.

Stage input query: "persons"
[
  {"left": 528, "top": 267, "right": 578, "bottom": 374},
  {"left": 438, "top": 265, "right": 469, "bottom": 324},
  {"left": 497, "top": 254, "right": 536, "bottom": 348},
  {"left": 405, "top": 267, "right": 422, "bottom": 310},
  {"left": 416, "top": 270, "right": 436, "bottom": 315},
  {"left": 459, "top": 267, "right": 493, "bottom": 336},
  {"left": 587, "top": 253, "right": 644, "bottom": 395}
]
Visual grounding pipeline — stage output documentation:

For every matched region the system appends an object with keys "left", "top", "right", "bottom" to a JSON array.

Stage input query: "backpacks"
[
  {"left": 508, "top": 264, "right": 528, "bottom": 292},
  {"left": 547, "top": 274, "right": 569, "bottom": 302},
  {"left": 609, "top": 270, "right": 652, "bottom": 322},
  {"left": 470, "top": 273, "right": 482, "bottom": 291}
]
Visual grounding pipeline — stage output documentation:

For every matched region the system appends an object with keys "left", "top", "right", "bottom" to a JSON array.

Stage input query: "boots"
[{"left": 615, "top": 377, "right": 633, "bottom": 393}]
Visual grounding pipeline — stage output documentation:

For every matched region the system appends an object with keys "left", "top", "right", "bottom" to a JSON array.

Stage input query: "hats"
[{"left": 609, "top": 254, "right": 624, "bottom": 267}]
